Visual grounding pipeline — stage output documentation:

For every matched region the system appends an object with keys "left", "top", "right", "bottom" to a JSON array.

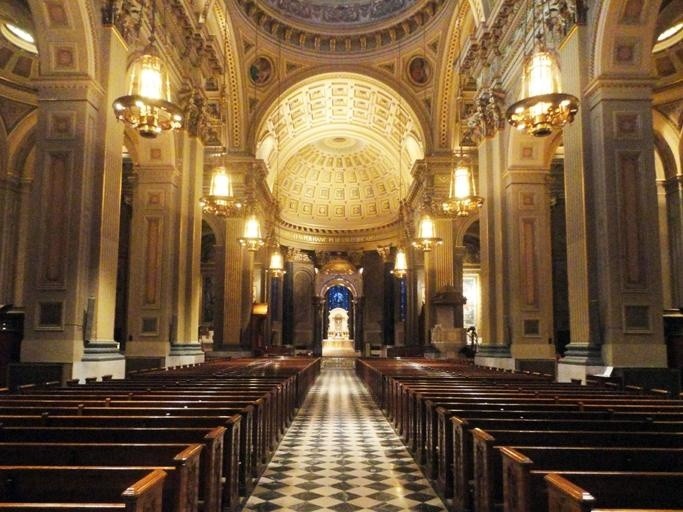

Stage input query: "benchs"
[
  {"left": 1, "top": 356, "right": 322, "bottom": 512},
  {"left": 352, "top": 355, "right": 680, "bottom": 510}
]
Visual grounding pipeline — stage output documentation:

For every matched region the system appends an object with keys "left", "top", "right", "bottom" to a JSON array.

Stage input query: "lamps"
[
  {"left": 111, "top": 0, "right": 185, "bottom": 139},
  {"left": 389, "top": 1, "right": 484, "bottom": 278},
  {"left": 503, "top": 0, "right": 581, "bottom": 138},
  {"left": 251, "top": 302, "right": 267, "bottom": 314},
  {"left": 196, "top": 0, "right": 287, "bottom": 279}
]
